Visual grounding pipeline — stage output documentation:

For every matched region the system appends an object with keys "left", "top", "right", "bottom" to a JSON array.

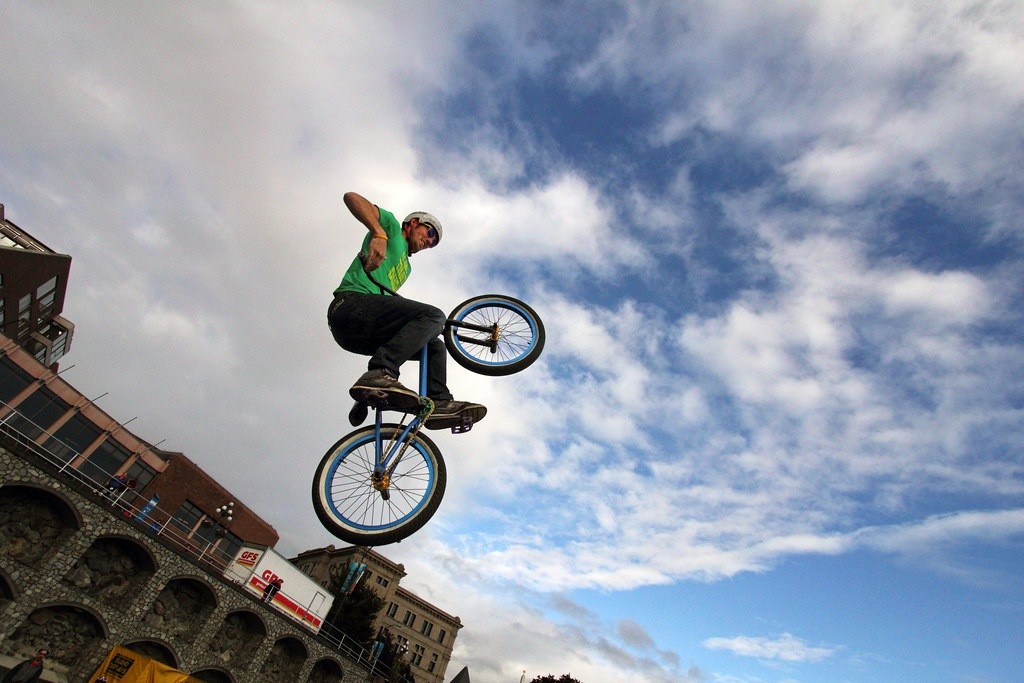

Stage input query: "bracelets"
[{"left": 372, "top": 234, "right": 388, "bottom": 239}]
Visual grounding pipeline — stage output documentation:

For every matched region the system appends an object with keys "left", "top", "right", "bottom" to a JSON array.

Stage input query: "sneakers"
[
  {"left": 424, "top": 400, "right": 486, "bottom": 429},
  {"left": 349, "top": 370, "right": 419, "bottom": 408}
]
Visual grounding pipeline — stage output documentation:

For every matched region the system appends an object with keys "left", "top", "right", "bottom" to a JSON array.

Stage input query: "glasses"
[
  {"left": 38, "top": 652, "right": 46, "bottom": 657},
  {"left": 421, "top": 222, "right": 437, "bottom": 248}
]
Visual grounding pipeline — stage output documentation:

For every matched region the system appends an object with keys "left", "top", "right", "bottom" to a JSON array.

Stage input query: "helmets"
[{"left": 402, "top": 212, "right": 443, "bottom": 244}]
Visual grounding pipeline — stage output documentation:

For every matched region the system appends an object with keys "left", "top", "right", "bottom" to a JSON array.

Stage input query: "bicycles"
[{"left": 311, "top": 252, "right": 546, "bottom": 546}]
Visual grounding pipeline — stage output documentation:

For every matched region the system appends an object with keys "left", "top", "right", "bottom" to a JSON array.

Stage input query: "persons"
[
  {"left": 261, "top": 579, "right": 284, "bottom": 604},
  {"left": 95, "top": 473, "right": 128, "bottom": 500},
  {"left": 326, "top": 192, "right": 487, "bottom": 430},
  {"left": 151, "top": 520, "right": 162, "bottom": 531}
]
